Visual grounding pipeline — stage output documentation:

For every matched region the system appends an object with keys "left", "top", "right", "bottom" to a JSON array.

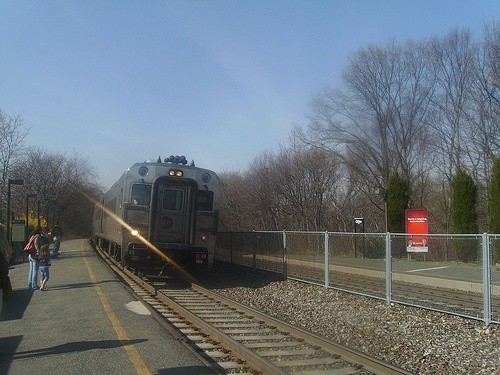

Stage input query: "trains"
[{"left": 92, "top": 156, "right": 222, "bottom": 273}]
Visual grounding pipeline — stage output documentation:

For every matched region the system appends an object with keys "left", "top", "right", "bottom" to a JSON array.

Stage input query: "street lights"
[
  {"left": 23, "top": 193, "right": 37, "bottom": 235},
  {"left": 5, "top": 179, "right": 24, "bottom": 243}
]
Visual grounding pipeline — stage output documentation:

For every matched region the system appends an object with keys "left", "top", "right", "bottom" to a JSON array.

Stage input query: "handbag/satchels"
[{"left": 24, "top": 241, "right": 37, "bottom": 254}]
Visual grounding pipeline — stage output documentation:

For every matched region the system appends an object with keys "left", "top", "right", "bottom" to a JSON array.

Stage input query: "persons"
[
  {"left": 28, "top": 225, "right": 43, "bottom": 291},
  {"left": 37, "top": 226, "right": 52, "bottom": 291},
  {"left": 0, "top": 222, "right": 11, "bottom": 313},
  {"left": 52, "top": 222, "right": 64, "bottom": 255}
]
